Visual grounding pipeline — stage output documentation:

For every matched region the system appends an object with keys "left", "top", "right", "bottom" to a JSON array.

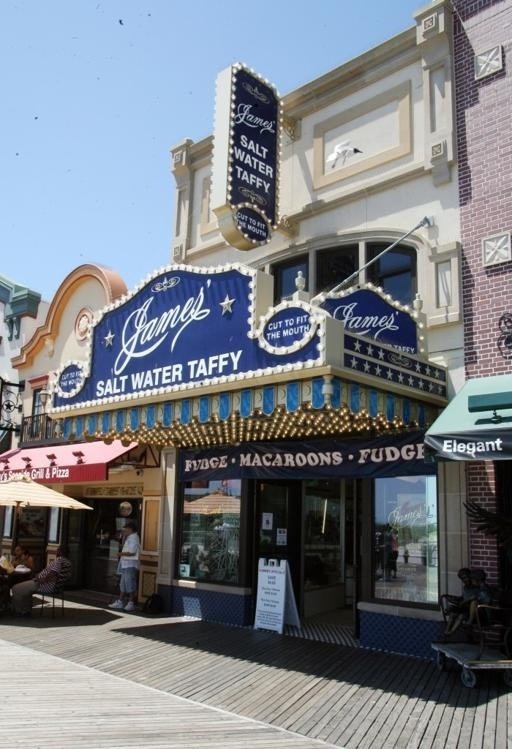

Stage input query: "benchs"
[{"left": 438, "top": 586, "right": 512, "bottom": 662}]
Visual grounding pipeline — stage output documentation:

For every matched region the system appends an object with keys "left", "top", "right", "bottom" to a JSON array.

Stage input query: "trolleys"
[{"left": 432, "top": 593, "right": 512, "bottom": 687}]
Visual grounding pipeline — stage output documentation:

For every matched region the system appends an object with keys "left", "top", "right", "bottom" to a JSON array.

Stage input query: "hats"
[{"left": 123, "top": 522, "right": 136, "bottom": 528}]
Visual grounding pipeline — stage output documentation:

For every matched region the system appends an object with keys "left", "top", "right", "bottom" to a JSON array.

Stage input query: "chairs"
[{"left": 32, "top": 560, "right": 74, "bottom": 619}]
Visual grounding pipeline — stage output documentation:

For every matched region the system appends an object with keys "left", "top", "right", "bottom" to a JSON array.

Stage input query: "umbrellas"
[{"left": 0, "top": 471, "right": 93, "bottom": 555}]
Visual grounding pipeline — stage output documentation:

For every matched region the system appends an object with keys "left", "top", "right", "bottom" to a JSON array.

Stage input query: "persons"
[
  {"left": 376, "top": 523, "right": 399, "bottom": 581},
  {"left": 108, "top": 523, "right": 140, "bottom": 611},
  {"left": 440, "top": 568, "right": 496, "bottom": 629},
  {"left": 11, "top": 544, "right": 71, "bottom": 616}
]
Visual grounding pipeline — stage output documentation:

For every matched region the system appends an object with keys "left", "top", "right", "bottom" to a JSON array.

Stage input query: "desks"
[{"left": 0, "top": 568, "right": 32, "bottom": 616}]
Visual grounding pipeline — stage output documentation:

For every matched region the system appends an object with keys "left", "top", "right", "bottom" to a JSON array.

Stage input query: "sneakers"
[{"left": 108, "top": 599, "right": 135, "bottom": 611}]
